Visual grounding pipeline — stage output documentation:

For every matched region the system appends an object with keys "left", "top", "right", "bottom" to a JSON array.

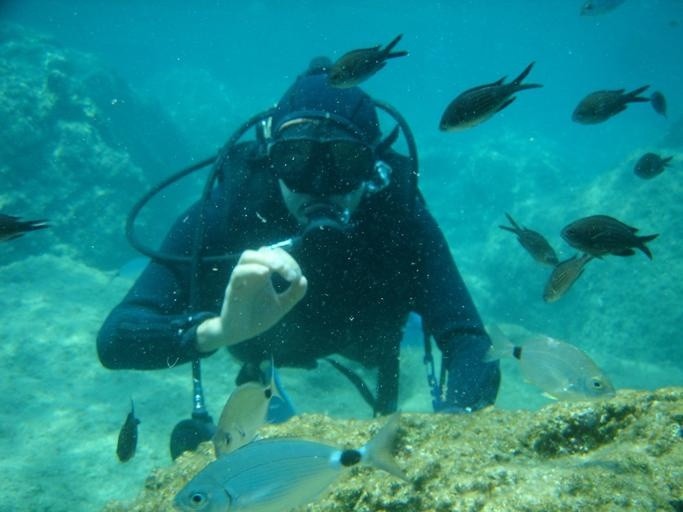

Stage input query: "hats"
[{"left": 271, "top": 74, "right": 381, "bottom": 142}]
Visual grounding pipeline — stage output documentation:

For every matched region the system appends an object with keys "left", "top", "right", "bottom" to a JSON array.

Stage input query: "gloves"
[{"left": 444, "top": 331, "right": 500, "bottom": 411}]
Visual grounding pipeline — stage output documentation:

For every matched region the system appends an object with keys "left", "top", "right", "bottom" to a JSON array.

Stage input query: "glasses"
[{"left": 270, "top": 138, "right": 374, "bottom": 195}]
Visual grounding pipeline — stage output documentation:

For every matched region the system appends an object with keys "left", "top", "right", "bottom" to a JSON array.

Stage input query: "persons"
[{"left": 91, "top": 54, "right": 503, "bottom": 420}]
